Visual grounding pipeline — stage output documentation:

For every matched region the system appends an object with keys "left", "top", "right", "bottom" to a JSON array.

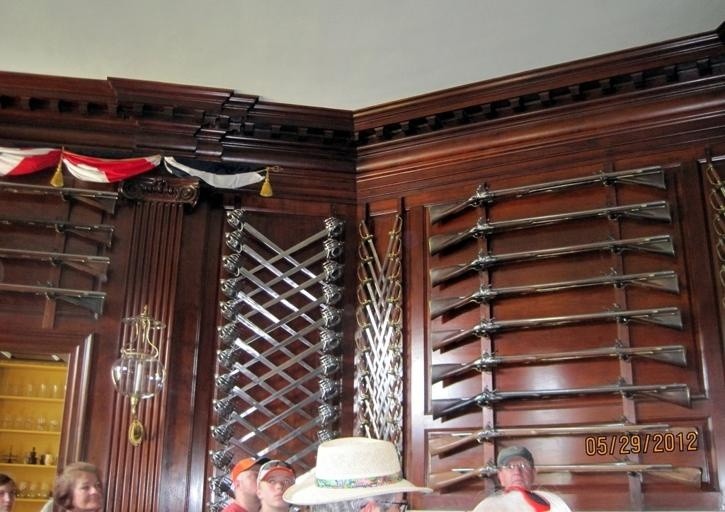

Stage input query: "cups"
[
  {"left": 0, "top": 413, "right": 59, "bottom": 432},
  {"left": 0, "top": 381, "right": 65, "bottom": 399},
  {"left": 45, "top": 453, "right": 57, "bottom": 466},
  {"left": 18, "top": 481, "right": 51, "bottom": 497}
]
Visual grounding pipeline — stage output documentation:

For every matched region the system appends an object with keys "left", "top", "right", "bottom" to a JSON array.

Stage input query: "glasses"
[
  {"left": 500, "top": 462, "right": 533, "bottom": 472},
  {"left": 357, "top": 499, "right": 410, "bottom": 512}
]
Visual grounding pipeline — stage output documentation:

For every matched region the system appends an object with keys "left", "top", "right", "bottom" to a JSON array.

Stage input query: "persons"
[
  {"left": 220, "top": 457, "right": 270, "bottom": 512},
  {"left": 472, "top": 484, "right": 573, "bottom": 511},
  {"left": 52, "top": 460, "right": 103, "bottom": 512},
  {"left": 495, "top": 446, "right": 536, "bottom": 490},
  {"left": 0, "top": 474, "right": 19, "bottom": 511},
  {"left": 256, "top": 458, "right": 295, "bottom": 511}
]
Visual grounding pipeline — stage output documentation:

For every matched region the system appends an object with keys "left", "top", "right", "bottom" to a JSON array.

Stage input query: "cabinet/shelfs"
[{"left": 0, "top": 358, "right": 69, "bottom": 512}]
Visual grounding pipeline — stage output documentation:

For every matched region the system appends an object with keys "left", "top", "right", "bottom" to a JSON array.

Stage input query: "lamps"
[{"left": 109, "top": 304, "right": 168, "bottom": 448}]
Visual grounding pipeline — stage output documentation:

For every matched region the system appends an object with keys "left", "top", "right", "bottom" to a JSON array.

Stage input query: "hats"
[
  {"left": 231, "top": 456, "right": 270, "bottom": 482},
  {"left": 256, "top": 460, "right": 297, "bottom": 487},
  {"left": 495, "top": 446, "right": 533, "bottom": 467}
]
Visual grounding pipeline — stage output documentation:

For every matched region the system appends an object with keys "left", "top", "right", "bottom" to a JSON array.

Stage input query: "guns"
[
  {"left": 429, "top": 464, "right": 674, "bottom": 490},
  {"left": 430, "top": 199, "right": 673, "bottom": 258},
  {"left": 430, "top": 166, "right": 666, "bottom": 228},
  {"left": 430, "top": 270, "right": 680, "bottom": 320},
  {"left": 0, "top": 177, "right": 118, "bottom": 315},
  {"left": 430, "top": 344, "right": 687, "bottom": 383},
  {"left": 428, "top": 233, "right": 675, "bottom": 288},
  {"left": 429, "top": 306, "right": 683, "bottom": 351},
  {"left": 452, "top": 467, "right": 702, "bottom": 490},
  {"left": 430, "top": 383, "right": 691, "bottom": 421},
  {"left": 430, "top": 424, "right": 699, "bottom": 456}
]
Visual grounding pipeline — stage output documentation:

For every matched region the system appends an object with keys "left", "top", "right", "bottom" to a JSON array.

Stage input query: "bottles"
[{"left": 26, "top": 446, "right": 44, "bottom": 465}]
